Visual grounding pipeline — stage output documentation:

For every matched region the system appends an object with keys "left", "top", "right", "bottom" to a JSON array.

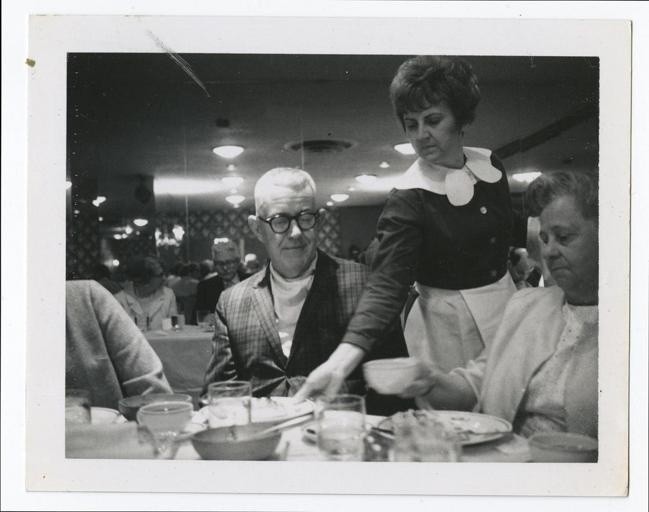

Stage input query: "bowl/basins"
[
  {"left": 119, "top": 393, "right": 193, "bottom": 419},
  {"left": 364, "top": 357, "right": 417, "bottom": 393},
  {"left": 193, "top": 424, "right": 281, "bottom": 459},
  {"left": 529, "top": 431, "right": 596, "bottom": 462}
]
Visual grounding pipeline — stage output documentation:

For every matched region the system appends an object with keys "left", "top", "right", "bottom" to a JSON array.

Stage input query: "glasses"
[
  {"left": 152, "top": 272, "right": 164, "bottom": 278},
  {"left": 213, "top": 259, "right": 237, "bottom": 266},
  {"left": 258, "top": 212, "right": 318, "bottom": 233}
]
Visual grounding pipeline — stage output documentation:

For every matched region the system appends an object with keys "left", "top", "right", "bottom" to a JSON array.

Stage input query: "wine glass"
[{"left": 140, "top": 401, "right": 193, "bottom": 458}]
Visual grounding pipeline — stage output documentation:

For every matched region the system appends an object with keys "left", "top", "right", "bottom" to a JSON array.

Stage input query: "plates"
[
  {"left": 88, "top": 406, "right": 120, "bottom": 425},
  {"left": 305, "top": 421, "right": 318, "bottom": 442},
  {"left": 195, "top": 397, "right": 315, "bottom": 427},
  {"left": 379, "top": 410, "right": 512, "bottom": 443}
]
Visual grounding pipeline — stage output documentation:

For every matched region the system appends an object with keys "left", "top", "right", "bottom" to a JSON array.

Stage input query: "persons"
[
  {"left": 293, "top": 56, "right": 532, "bottom": 416},
  {"left": 506, "top": 247, "right": 537, "bottom": 288},
  {"left": 399, "top": 167, "right": 597, "bottom": 441},
  {"left": 92, "top": 236, "right": 374, "bottom": 330},
  {"left": 198, "top": 166, "right": 419, "bottom": 417},
  {"left": 67, "top": 278, "right": 176, "bottom": 409}
]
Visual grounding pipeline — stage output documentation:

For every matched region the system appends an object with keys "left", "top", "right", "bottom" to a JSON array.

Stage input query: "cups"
[
  {"left": 209, "top": 381, "right": 252, "bottom": 426},
  {"left": 65, "top": 389, "right": 92, "bottom": 424},
  {"left": 197, "top": 310, "right": 209, "bottom": 329},
  {"left": 162, "top": 318, "right": 171, "bottom": 330},
  {"left": 387, "top": 420, "right": 464, "bottom": 462},
  {"left": 172, "top": 312, "right": 185, "bottom": 335},
  {"left": 316, "top": 394, "right": 367, "bottom": 459}
]
